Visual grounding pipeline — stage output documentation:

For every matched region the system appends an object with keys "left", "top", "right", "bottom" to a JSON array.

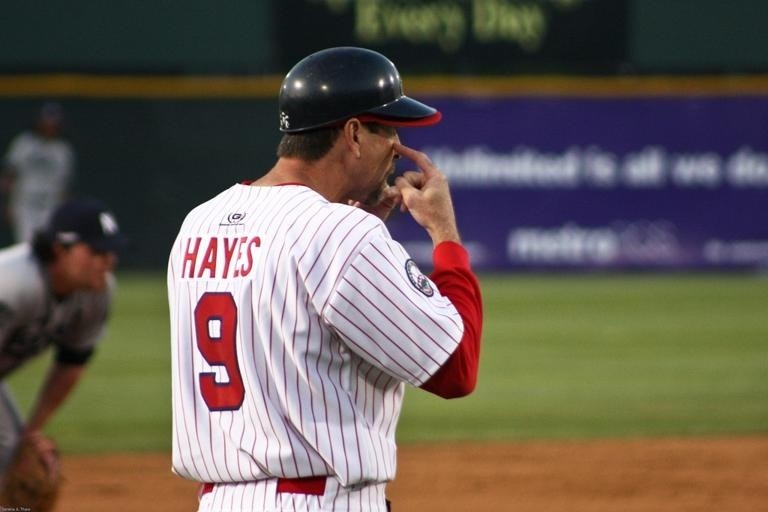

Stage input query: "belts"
[{"left": 197, "top": 475, "right": 328, "bottom": 499}]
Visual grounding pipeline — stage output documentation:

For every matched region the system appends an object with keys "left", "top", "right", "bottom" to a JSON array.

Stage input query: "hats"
[
  {"left": 50, "top": 196, "right": 128, "bottom": 253},
  {"left": 277, "top": 47, "right": 444, "bottom": 138}
]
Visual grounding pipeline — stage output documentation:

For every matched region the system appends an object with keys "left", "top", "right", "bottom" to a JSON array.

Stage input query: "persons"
[
  {"left": 0, "top": 194, "right": 138, "bottom": 510},
  {"left": 167, "top": 44, "right": 484, "bottom": 512},
  {"left": 0, "top": 101, "right": 81, "bottom": 252}
]
